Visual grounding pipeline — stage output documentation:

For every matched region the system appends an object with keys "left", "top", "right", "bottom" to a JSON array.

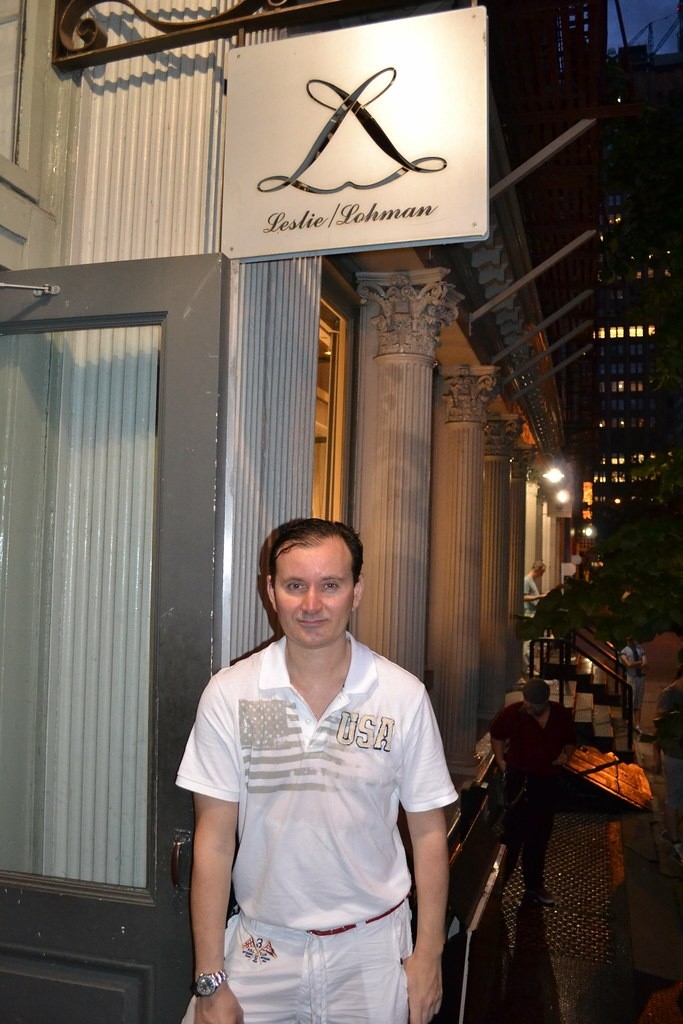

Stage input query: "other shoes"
[
  {"left": 660, "top": 829, "right": 683, "bottom": 847},
  {"left": 670, "top": 844, "right": 683, "bottom": 867},
  {"left": 636, "top": 726, "right": 647, "bottom": 736},
  {"left": 526, "top": 881, "right": 557, "bottom": 906}
]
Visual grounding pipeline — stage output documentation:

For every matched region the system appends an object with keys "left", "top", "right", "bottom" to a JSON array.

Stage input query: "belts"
[{"left": 302, "top": 890, "right": 412, "bottom": 937}]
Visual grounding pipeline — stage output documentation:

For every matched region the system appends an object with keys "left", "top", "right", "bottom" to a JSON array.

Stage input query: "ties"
[{"left": 632, "top": 647, "right": 642, "bottom": 678}]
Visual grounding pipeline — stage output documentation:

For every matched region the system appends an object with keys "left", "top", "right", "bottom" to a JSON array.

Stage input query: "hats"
[{"left": 522, "top": 677, "right": 550, "bottom": 703}]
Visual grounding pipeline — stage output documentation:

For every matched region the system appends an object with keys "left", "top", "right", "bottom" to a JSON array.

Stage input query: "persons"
[
  {"left": 175, "top": 518, "right": 458, "bottom": 1023},
  {"left": 490, "top": 679, "right": 578, "bottom": 903},
  {"left": 622, "top": 637, "right": 648, "bottom": 735},
  {"left": 524, "top": 562, "right": 548, "bottom": 675},
  {"left": 656, "top": 664, "right": 683, "bottom": 865}
]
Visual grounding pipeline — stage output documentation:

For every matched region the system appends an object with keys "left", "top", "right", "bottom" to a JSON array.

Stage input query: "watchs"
[{"left": 192, "top": 968, "right": 226, "bottom": 997}]
